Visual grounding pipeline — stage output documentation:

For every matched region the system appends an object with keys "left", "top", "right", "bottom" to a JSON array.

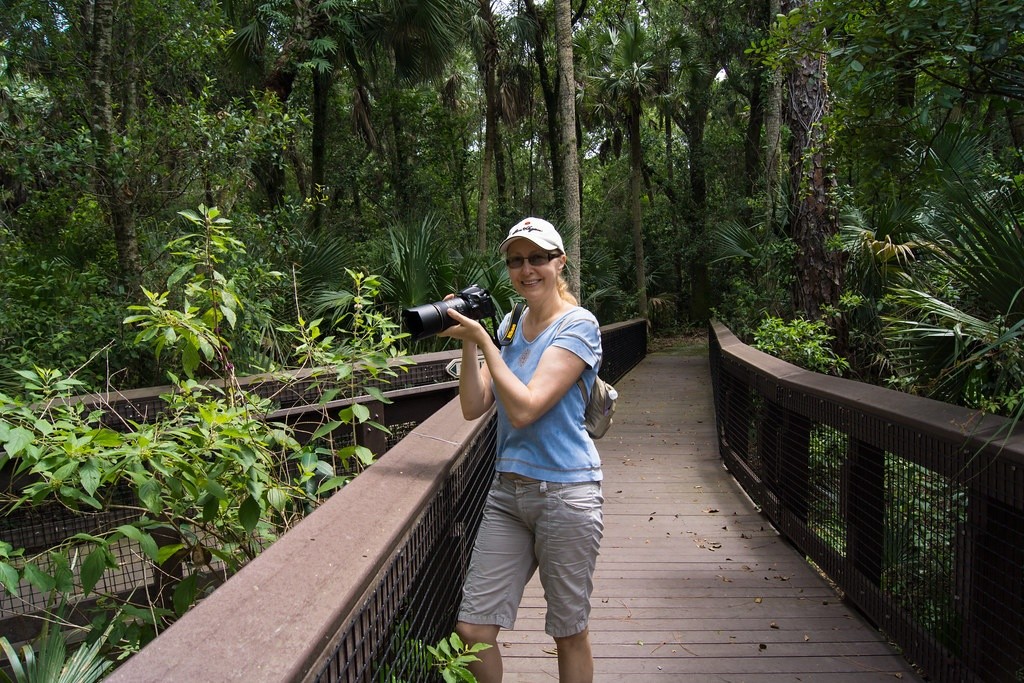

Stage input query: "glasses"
[{"left": 505, "top": 251, "right": 561, "bottom": 269}]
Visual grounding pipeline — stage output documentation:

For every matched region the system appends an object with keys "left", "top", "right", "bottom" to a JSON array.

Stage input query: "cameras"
[{"left": 401, "top": 284, "right": 499, "bottom": 341}]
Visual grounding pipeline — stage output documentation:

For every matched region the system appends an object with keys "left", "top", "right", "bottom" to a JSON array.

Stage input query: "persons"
[{"left": 439, "top": 216, "right": 604, "bottom": 683}]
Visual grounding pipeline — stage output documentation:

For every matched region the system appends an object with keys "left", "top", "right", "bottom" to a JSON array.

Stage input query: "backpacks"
[{"left": 557, "top": 310, "right": 617, "bottom": 439}]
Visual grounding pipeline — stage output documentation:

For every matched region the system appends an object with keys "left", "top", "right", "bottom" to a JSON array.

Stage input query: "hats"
[{"left": 499, "top": 216, "right": 566, "bottom": 256}]
